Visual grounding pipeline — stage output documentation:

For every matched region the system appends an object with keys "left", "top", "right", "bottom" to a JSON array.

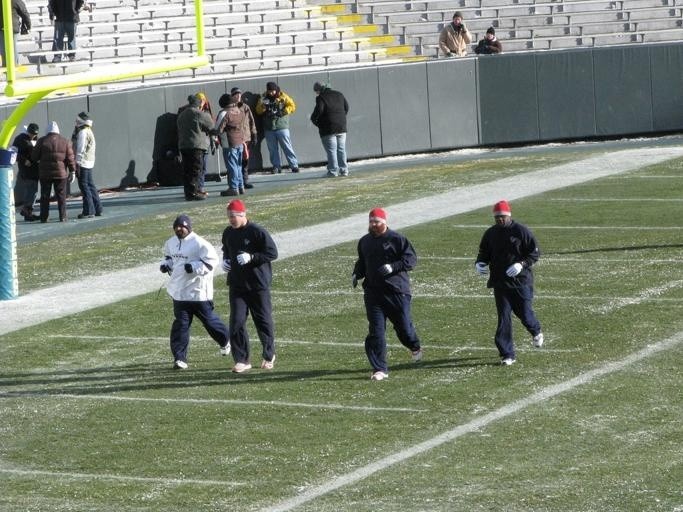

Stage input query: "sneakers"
[
  {"left": 533, "top": 333, "right": 543, "bottom": 347},
  {"left": 371, "top": 371, "right": 388, "bottom": 381},
  {"left": 21, "top": 209, "right": 68, "bottom": 222},
  {"left": 78, "top": 214, "right": 95, "bottom": 218},
  {"left": 187, "top": 191, "right": 209, "bottom": 201},
  {"left": 292, "top": 167, "right": 298, "bottom": 172},
  {"left": 501, "top": 357, "right": 515, "bottom": 365},
  {"left": 261, "top": 354, "right": 275, "bottom": 369},
  {"left": 220, "top": 338, "right": 231, "bottom": 355},
  {"left": 174, "top": 360, "right": 187, "bottom": 369},
  {"left": 323, "top": 171, "right": 335, "bottom": 177},
  {"left": 232, "top": 362, "right": 252, "bottom": 373},
  {"left": 272, "top": 168, "right": 281, "bottom": 173},
  {"left": 412, "top": 348, "right": 421, "bottom": 361},
  {"left": 221, "top": 184, "right": 253, "bottom": 196}
]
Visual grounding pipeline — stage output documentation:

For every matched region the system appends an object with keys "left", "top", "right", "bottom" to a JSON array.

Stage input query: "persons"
[
  {"left": 176, "top": 88, "right": 255, "bottom": 200},
  {"left": 13, "top": 123, "right": 41, "bottom": 220},
  {"left": 158, "top": 216, "right": 230, "bottom": 369},
  {"left": 219, "top": 200, "right": 278, "bottom": 373},
  {"left": 32, "top": 120, "right": 76, "bottom": 224},
  {"left": 69, "top": 111, "right": 102, "bottom": 219},
  {"left": 48, "top": 1, "right": 83, "bottom": 62},
  {"left": 439, "top": 11, "right": 472, "bottom": 57},
  {"left": 256, "top": 82, "right": 299, "bottom": 173},
  {"left": 310, "top": 81, "right": 350, "bottom": 177},
  {"left": 475, "top": 26, "right": 502, "bottom": 54},
  {"left": 353, "top": 208, "right": 422, "bottom": 381},
  {"left": 474, "top": 201, "right": 545, "bottom": 367},
  {"left": 0, "top": 0, "right": 32, "bottom": 68}
]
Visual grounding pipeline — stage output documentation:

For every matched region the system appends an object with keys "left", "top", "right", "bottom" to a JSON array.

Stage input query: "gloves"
[
  {"left": 476, "top": 262, "right": 488, "bottom": 274},
  {"left": 160, "top": 265, "right": 169, "bottom": 272},
  {"left": 353, "top": 274, "right": 357, "bottom": 288},
  {"left": 75, "top": 167, "right": 80, "bottom": 177},
  {"left": 379, "top": 264, "right": 393, "bottom": 276},
  {"left": 185, "top": 264, "right": 192, "bottom": 273},
  {"left": 237, "top": 251, "right": 254, "bottom": 266},
  {"left": 506, "top": 263, "right": 523, "bottom": 277},
  {"left": 68, "top": 171, "right": 75, "bottom": 184},
  {"left": 222, "top": 259, "right": 232, "bottom": 272}
]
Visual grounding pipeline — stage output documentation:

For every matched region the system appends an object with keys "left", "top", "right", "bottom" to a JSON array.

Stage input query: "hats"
[
  {"left": 174, "top": 215, "right": 192, "bottom": 232},
  {"left": 492, "top": 201, "right": 512, "bottom": 217},
  {"left": 27, "top": 123, "right": 39, "bottom": 134},
  {"left": 48, "top": 121, "right": 60, "bottom": 134},
  {"left": 227, "top": 200, "right": 246, "bottom": 217},
  {"left": 267, "top": 82, "right": 276, "bottom": 90},
  {"left": 219, "top": 88, "right": 241, "bottom": 107},
  {"left": 314, "top": 81, "right": 325, "bottom": 91},
  {"left": 188, "top": 93, "right": 206, "bottom": 107},
  {"left": 77, "top": 112, "right": 88, "bottom": 122},
  {"left": 369, "top": 208, "right": 386, "bottom": 224}
]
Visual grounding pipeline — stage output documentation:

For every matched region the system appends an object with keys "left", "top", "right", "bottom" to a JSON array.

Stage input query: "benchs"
[{"left": 0, "top": 0, "right": 683, "bottom": 105}]
